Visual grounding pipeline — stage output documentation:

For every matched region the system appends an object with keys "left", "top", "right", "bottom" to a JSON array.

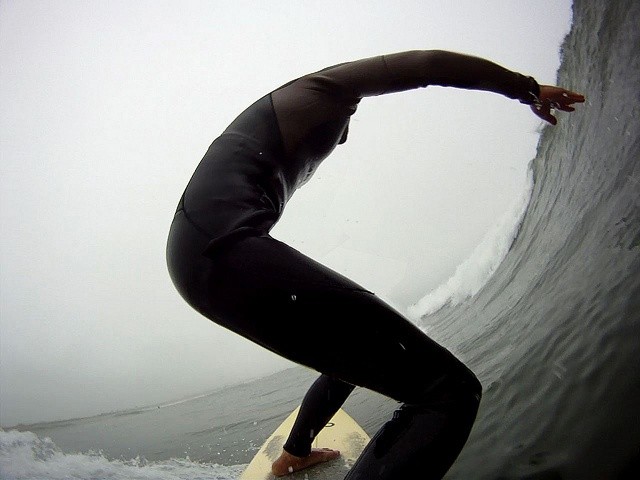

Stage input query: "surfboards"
[{"left": 240, "top": 405, "right": 372, "bottom": 480}]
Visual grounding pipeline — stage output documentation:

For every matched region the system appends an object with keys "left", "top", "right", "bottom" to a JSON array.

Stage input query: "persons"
[{"left": 164, "top": 49, "right": 586, "bottom": 480}]
